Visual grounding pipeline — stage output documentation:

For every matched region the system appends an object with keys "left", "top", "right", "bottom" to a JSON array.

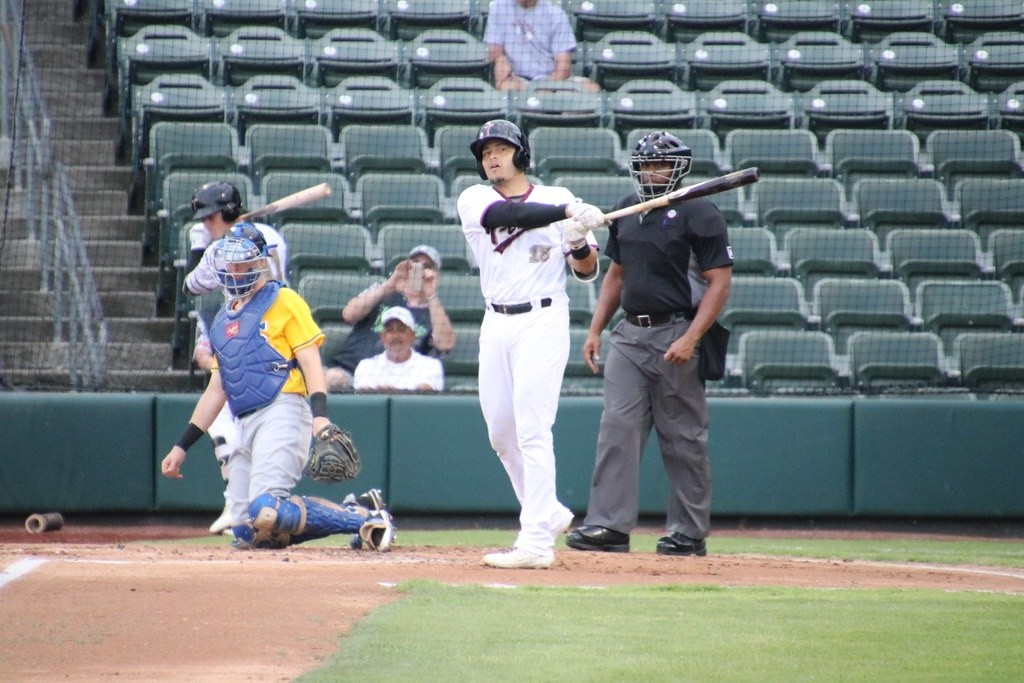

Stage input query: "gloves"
[
  {"left": 566, "top": 220, "right": 586, "bottom": 250},
  {"left": 568, "top": 197, "right": 613, "bottom": 234}
]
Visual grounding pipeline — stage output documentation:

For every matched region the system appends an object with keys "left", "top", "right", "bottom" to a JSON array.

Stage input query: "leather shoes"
[
  {"left": 565, "top": 525, "right": 630, "bottom": 553},
  {"left": 657, "top": 531, "right": 707, "bottom": 556}
]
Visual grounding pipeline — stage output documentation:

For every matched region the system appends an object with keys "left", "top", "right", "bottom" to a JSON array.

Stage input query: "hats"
[
  {"left": 382, "top": 306, "right": 415, "bottom": 331},
  {"left": 408, "top": 245, "right": 442, "bottom": 270}
]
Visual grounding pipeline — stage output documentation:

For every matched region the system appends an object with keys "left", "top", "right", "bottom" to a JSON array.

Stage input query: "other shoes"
[{"left": 209, "top": 505, "right": 235, "bottom": 535}]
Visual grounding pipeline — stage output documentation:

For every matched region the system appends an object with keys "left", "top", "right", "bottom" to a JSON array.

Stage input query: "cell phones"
[{"left": 408, "top": 263, "right": 423, "bottom": 292}]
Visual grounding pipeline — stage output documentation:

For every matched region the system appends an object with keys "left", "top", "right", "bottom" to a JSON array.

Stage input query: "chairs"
[
  {"left": 245, "top": 123, "right": 333, "bottom": 196},
  {"left": 100, "top": 0, "right": 380, "bottom": 117},
  {"left": 170, "top": 222, "right": 202, "bottom": 370},
  {"left": 384, "top": 0, "right": 1024, "bottom": 388},
  {"left": 297, "top": 273, "right": 388, "bottom": 329},
  {"left": 154, "top": 173, "right": 251, "bottom": 317},
  {"left": 126, "top": 73, "right": 231, "bottom": 215},
  {"left": 261, "top": 172, "right": 351, "bottom": 232},
  {"left": 114, "top": 25, "right": 213, "bottom": 166},
  {"left": 325, "top": 74, "right": 419, "bottom": 142},
  {"left": 139, "top": 122, "right": 237, "bottom": 269},
  {"left": 278, "top": 223, "right": 372, "bottom": 294},
  {"left": 339, "top": 124, "right": 427, "bottom": 193},
  {"left": 357, "top": 174, "right": 443, "bottom": 244},
  {"left": 378, "top": 224, "right": 471, "bottom": 278},
  {"left": 318, "top": 323, "right": 354, "bottom": 365},
  {"left": 231, "top": 73, "right": 325, "bottom": 144}
]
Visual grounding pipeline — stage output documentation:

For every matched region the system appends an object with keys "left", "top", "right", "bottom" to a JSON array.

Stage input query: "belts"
[
  {"left": 626, "top": 311, "right": 688, "bottom": 328},
  {"left": 487, "top": 298, "right": 552, "bottom": 314}
]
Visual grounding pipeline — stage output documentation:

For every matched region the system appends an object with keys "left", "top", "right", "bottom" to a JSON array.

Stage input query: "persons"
[
  {"left": 158, "top": 221, "right": 398, "bottom": 554},
  {"left": 181, "top": 182, "right": 286, "bottom": 538},
  {"left": 564, "top": 132, "right": 734, "bottom": 555},
  {"left": 321, "top": 243, "right": 455, "bottom": 393},
  {"left": 457, "top": 120, "right": 613, "bottom": 571},
  {"left": 484, "top": 0, "right": 601, "bottom": 96}
]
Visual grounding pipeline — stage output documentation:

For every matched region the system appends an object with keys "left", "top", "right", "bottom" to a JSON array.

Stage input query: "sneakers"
[
  {"left": 351, "top": 489, "right": 397, "bottom": 552},
  {"left": 482, "top": 546, "right": 552, "bottom": 570},
  {"left": 553, "top": 515, "right": 575, "bottom": 542}
]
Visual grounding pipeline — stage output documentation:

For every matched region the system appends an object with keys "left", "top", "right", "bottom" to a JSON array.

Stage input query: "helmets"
[
  {"left": 470, "top": 119, "right": 531, "bottom": 180},
  {"left": 626, "top": 131, "right": 693, "bottom": 204},
  {"left": 209, "top": 221, "right": 278, "bottom": 302},
  {"left": 192, "top": 182, "right": 242, "bottom": 223}
]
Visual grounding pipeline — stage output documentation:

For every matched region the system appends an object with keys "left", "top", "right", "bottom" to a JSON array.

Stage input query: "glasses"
[{"left": 411, "top": 260, "right": 434, "bottom": 269}]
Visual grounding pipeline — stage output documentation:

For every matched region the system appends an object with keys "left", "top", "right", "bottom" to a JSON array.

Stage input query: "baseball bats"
[
  {"left": 603, "top": 166, "right": 761, "bottom": 221},
  {"left": 235, "top": 182, "right": 332, "bottom": 222}
]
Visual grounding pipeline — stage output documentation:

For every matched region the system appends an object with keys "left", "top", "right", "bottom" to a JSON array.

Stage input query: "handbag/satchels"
[{"left": 688, "top": 307, "right": 731, "bottom": 380}]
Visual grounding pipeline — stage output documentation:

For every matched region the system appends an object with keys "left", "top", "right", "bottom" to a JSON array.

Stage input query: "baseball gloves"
[{"left": 301, "top": 424, "right": 362, "bottom": 484}]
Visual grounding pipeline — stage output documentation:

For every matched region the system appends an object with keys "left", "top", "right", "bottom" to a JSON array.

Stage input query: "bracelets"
[{"left": 423, "top": 293, "right": 438, "bottom": 303}]
